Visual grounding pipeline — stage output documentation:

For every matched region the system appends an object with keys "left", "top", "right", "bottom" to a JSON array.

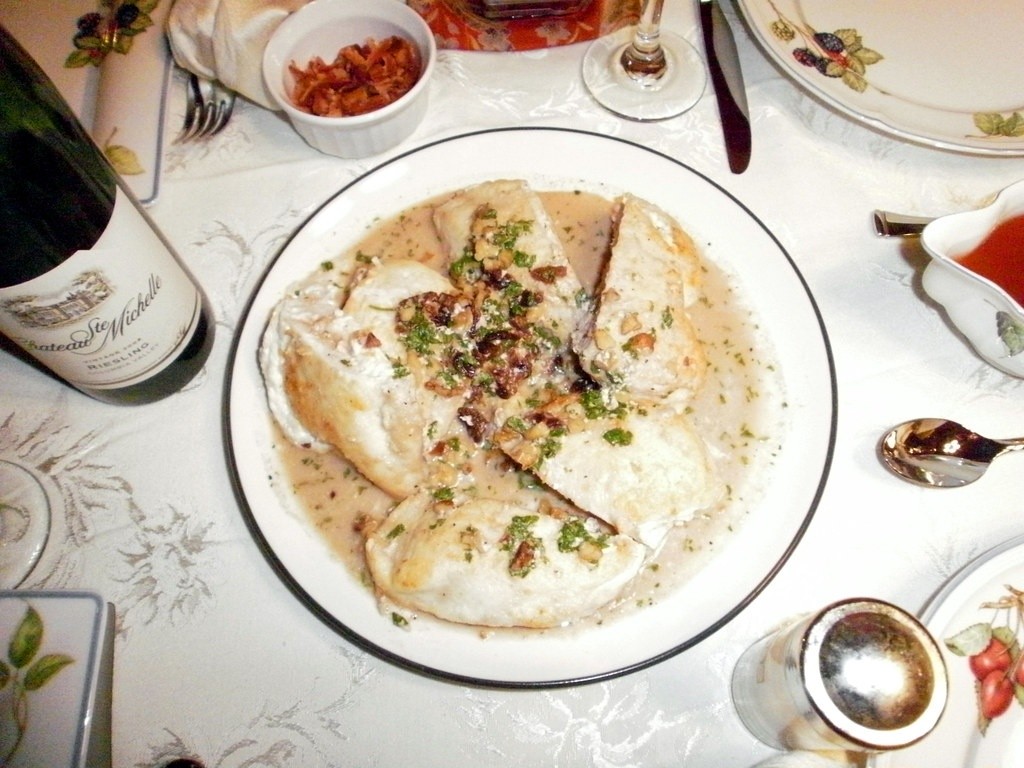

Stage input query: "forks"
[{"left": 171, "top": 71, "right": 236, "bottom": 146}]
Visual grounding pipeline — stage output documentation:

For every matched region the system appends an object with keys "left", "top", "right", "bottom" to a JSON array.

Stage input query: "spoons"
[{"left": 881, "top": 419, "right": 1024, "bottom": 490}]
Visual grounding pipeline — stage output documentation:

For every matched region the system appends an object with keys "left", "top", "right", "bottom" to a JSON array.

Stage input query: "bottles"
[{"left": 0, "top": 21, "right": 216, "bottom": 408}]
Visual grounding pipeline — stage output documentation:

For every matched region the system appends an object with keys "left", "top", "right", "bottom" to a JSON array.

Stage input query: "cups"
[
  {"left": 453, "top": 0, "right": 595, "bottom": 27},
  {"left": 730, "top": 595, "right": 949, "bottom": 768}
]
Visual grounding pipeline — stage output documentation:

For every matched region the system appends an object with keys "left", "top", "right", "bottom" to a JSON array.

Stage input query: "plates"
[
  {"left": 735, "top": 0, "right": 1024, "bottom": 157},
  {"left": 863, "top": 535, "right": 1024, "bottom": 768},
  {"left": 0, "top": 590, "right": 108, "bottom": 768},
  {"left": 220, "top": 125, "right": 838, "bottom": 692}
]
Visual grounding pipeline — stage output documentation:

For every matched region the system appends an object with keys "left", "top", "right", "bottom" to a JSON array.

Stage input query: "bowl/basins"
[
  {"left": 920, "top": 176, "right": 1024, "bottom": 380},
  {"left": 261, "top": 0, "right": 438, "bottom": 159}
]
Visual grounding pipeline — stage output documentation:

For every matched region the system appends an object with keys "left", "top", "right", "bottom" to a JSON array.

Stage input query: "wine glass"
[{"left": 582, "top": 0, "right": 708, "bottom": 123}]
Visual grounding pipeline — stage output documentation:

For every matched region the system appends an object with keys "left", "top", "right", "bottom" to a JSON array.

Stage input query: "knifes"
[{"left": 698, "top": 0, "right": 752, "bottom": 174}]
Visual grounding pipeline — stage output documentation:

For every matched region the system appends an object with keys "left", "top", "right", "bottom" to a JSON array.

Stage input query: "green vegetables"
[{"left": 308, "top": 206, "right": 634, "bottom": 571}]
